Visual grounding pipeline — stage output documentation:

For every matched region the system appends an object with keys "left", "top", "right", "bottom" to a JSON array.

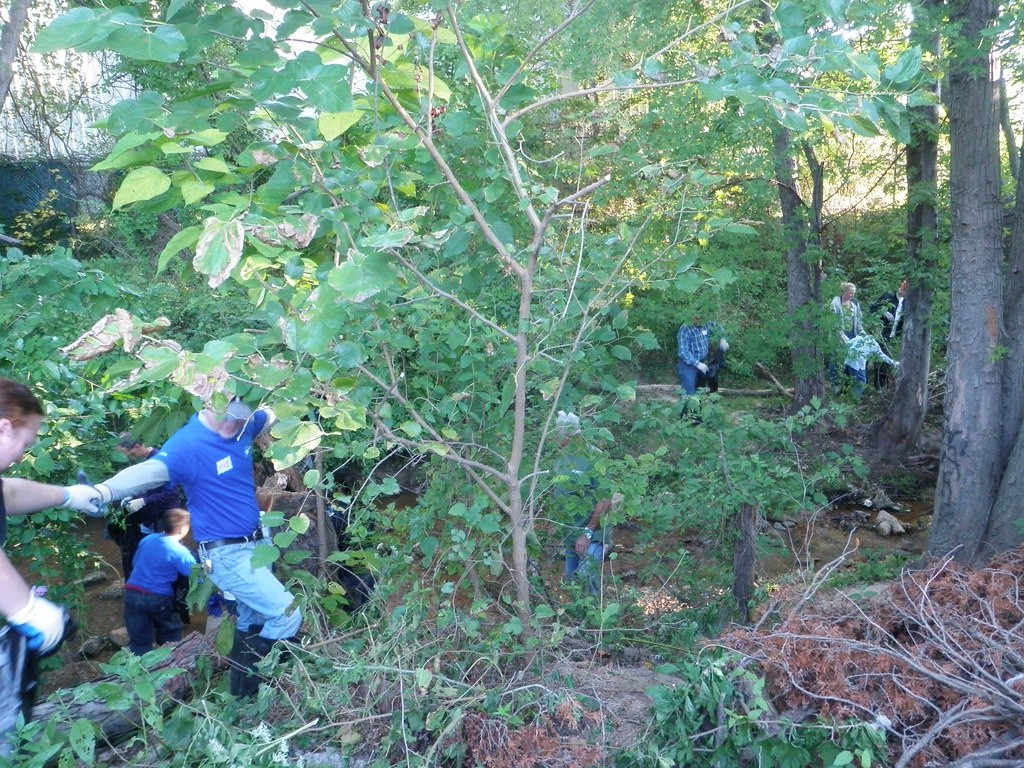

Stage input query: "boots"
[
  {"left": 246, "top": 636, "right": 295, "bottom": 697},
  {"left": 231, "top": 624, "right": 264, "bottom": 701}
]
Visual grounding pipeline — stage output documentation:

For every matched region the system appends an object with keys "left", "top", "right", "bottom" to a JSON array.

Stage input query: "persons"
[
  {"left": 838, "top": 322, "right": 899, "bottom": 402},
  {"left": 303, "top": 448, "right": 378, "bottom": 617},
  {"left": 124, "top": 508, "right": 224, "bottom": 657},
  {"left": 551, "top": 410, "right": 613, "bottom": 600},
  {"left": 677, "top": 307, "right": 730, "bottom": 427},
  {"left": 106, "top": 430, "right": 191, "bottom": 627},
  {"left": 75, "top": 390, "right": 304, "bottom": 702},
  {"left": 869, "top": 278, "right": 908, "bottom": 390},
  {"left": 829, "top": 282, "right": 867, "bottom": 398},
  {"left": 0, "top": 379, "right": 103, "bottom": 768}
]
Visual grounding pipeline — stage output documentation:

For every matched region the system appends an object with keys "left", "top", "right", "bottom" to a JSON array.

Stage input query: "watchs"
[{"left": 583, "top": 532, "right": 592, "bottom": 540}]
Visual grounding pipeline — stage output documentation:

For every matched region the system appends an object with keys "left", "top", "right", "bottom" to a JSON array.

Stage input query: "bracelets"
[{"left": 585, "top": 526, "right": 594, "bottom": 534}]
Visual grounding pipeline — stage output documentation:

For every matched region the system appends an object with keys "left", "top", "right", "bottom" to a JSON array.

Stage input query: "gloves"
[
  {"left": 894, "top": 362, "right": 899, "bottom": 368},
  {"left": 719, "top": 339, "right": 730, "bottom": 354},
  {"left": 884, "top": 311, "right": 895, "bottom": 322},
  {"left": 120, "top": 496, "right": 133, "bottom": 507},
  {"left": 127, "top": 498, "right": 146, "bottom": 513},
  {"left": 859, "top": 330, "right": 866, "bottom": 335},
  {"left": 77, "top": 471, "right": 112, "bottom": 517},
  {"left": 6, "top": 590, "right": 65, "bottom": 656},
  {"left": 54, "top": 484, "right": 102, "bottom": 512},
  {"left": 696, "top": 362, "right": 710, "bottom": 375},
  {"left": 841, "top": 334, "right": 850, "bottom": 342}
]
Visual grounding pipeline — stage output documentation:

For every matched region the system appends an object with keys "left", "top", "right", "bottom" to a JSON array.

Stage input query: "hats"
[{"left": 225, "top": 396, "right": 254, "bottom": 422}]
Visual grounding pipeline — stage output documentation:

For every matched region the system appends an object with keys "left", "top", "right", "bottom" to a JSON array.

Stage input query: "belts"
[{"left": 197, "top": 530, "right": 263, "bottom": 550}]
